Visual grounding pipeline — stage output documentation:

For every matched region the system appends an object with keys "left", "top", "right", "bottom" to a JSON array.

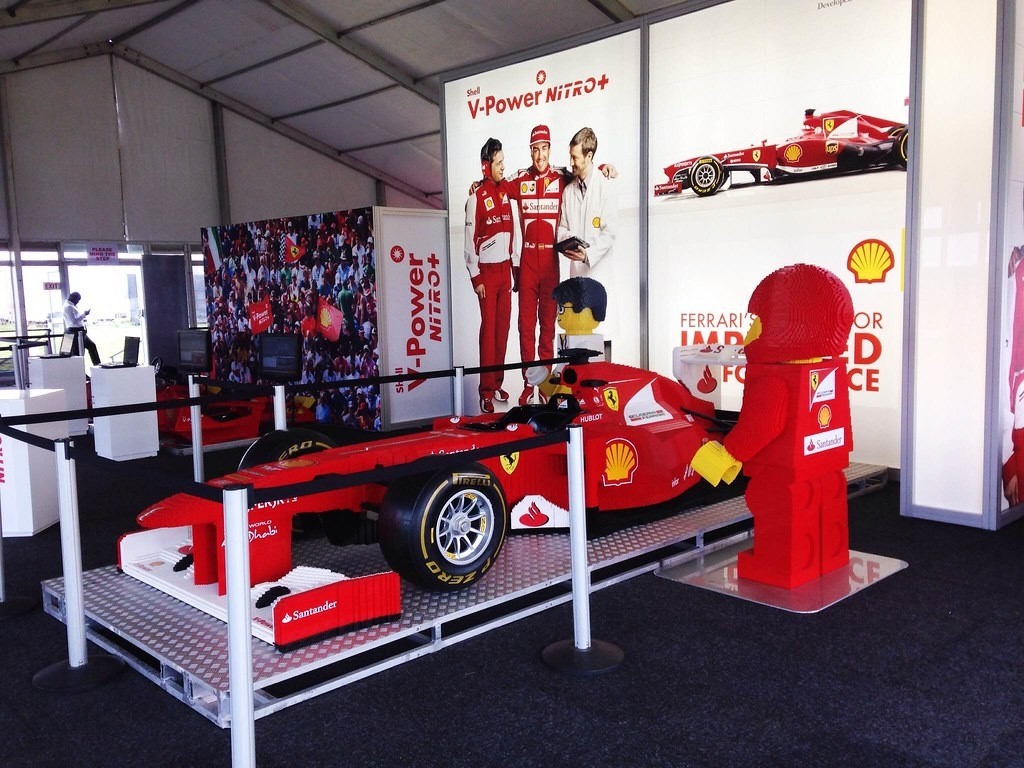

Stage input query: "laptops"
[
  {"left": 98, "top": 337, "right": 140, "bottom": 368},
  {"left": 40, "top": 332, "right": 77, "bottom": 359}
]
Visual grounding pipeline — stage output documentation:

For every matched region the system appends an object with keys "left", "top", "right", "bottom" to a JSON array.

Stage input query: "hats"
[{"left": 529, "top": 124, "right": 551, "bottom": 149}]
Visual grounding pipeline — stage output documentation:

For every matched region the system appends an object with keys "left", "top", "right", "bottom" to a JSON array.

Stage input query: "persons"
[
  {"left": 557, "top": 127, "right": 617, "bottom": 363},
  {"left": 62, "top": 292, "right": 100, "bottom": 366},
  {"left": 1003, "top": 244, "right": 1024, "bottom": 506},
  {"left": 524, "top": 277, "right": 607, "bottom": 400},
  {"left": 690, "top": 264, "right": 854, "bottom": 590},
  {"left": 469, "top": 125, "right": 618, "bottom": 406},
  {"left": 464, "top": 137, "right": 524, "bottom": 414},
  {"left": 202, "top": 208, "right": 381, "bottom": 432}
]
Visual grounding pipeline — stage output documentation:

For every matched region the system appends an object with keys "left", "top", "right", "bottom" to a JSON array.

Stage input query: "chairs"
[{"left": 497, "top": 393, "right": 587, "bottom": 433}]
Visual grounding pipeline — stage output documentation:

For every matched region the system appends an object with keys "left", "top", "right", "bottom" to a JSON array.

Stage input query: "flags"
[
  {"left": 281, "top": 236, "right": 305, "bottom": 263},
  {"left": 248, "top": 294, "right": 274, "bottom": 334},
  {"left": 315, "top": 298, "right": 343, "bottom": 342}
]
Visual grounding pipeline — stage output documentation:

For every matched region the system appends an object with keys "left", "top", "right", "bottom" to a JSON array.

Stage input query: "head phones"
[{"left": 481, "top": 137, "right": 494, "bottom": 178}]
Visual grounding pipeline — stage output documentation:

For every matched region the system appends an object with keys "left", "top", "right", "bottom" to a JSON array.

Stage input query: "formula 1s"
[
  {"left": 651, "top": 107, "right": 910, "bottom": 201},
  {"left": 115, "top": 347, "right": 729, "bottom": 654}
]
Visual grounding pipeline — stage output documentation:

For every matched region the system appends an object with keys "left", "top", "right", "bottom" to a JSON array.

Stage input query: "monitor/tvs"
[
  {"left": 258, "top": 333, "right": 304, "bottom": 381},
  {"left": 177, "top": 331, "right": 212, "bottom": 373}
]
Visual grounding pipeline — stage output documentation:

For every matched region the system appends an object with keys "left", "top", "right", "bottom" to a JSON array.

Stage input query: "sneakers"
[
  {"left": 539, "top": 388, "right": 548, "bottom": 405},
  {"left": 494, "top": 389, "right": 509, "bottom": 402},
  {"left": 480, "top": 398, "right": 494, "bottom": 413},
  {"left": 519, "top": 384, "right": 533, "bottom": 405}
]
